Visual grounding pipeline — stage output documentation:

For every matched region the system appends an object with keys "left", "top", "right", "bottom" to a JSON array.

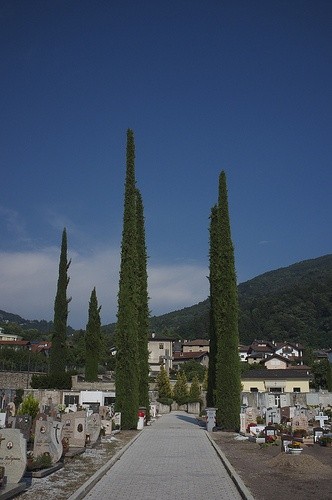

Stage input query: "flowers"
[
  {"left": 26, "top": 451, "right": 35, "bottom": 459},
  {"left": 246, "top": 422, "right": 332, "bottom": 448}
]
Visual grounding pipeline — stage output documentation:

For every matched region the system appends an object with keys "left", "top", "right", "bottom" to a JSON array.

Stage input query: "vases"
[
  {"left": 27, "top": 459, "right": 33, "bottom": 464},
  {"left": 289, "top": 448, "right": 303, "bottom": 455}
]
